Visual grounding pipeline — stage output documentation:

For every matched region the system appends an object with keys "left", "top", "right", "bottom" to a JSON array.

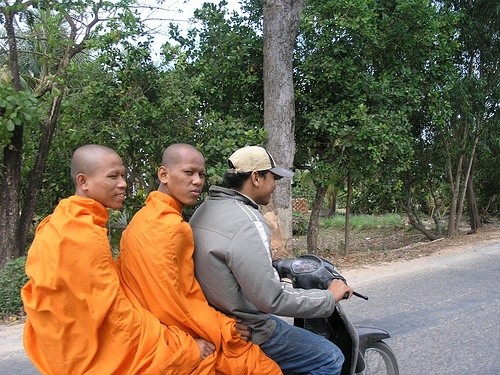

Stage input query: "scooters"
[{"left": 272, "top": 253, "right": 401, "bottom": 375}]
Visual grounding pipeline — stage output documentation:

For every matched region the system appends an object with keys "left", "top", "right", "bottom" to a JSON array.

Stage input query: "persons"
[
  {"left": 188, "top": 145, "right": 351, "bottom": 374},
  {"left": 116, "top": 143, "right": 284, "bottom": 374},
  {"left": 20, "top": 144, "right": 216, "bottom": 374}
]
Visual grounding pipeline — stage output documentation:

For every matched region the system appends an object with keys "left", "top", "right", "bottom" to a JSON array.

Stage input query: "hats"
[{"left": 226, "top": 144, "right": 296, "bottom": 180}]
